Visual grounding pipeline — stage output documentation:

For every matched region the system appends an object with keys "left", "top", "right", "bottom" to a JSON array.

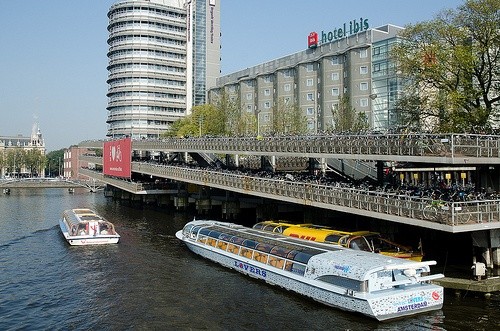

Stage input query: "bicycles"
[{"left": 130, "top": 156, "right": 500, "bottom": 224}]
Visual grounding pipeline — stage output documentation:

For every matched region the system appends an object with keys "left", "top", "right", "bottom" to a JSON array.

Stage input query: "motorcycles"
[{"left": 134, "top": 125, "right": 500, "bottom": 154}]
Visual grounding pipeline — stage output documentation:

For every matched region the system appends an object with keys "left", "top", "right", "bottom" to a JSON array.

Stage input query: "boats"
[
  {"left": 251, "top": 220, "right": 423, "bottom": 262},
  {"left": 176, "top": 216, "right": 443, "bottom": 322},
  {"left": 58, "top": 208, "right": 121, "bottom": 245}
]
